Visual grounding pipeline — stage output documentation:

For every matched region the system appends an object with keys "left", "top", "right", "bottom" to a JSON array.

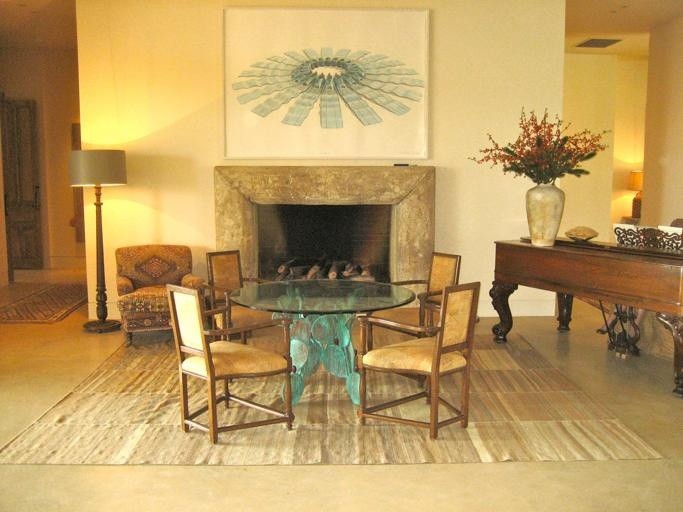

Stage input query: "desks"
[{"left": 488, "top": 239, "right": 682, "bottom": 397}]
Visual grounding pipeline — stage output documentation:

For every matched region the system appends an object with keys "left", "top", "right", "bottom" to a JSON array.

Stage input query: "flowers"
[{"left": 466, "top": 106, "right": 612, "bottom": 186}]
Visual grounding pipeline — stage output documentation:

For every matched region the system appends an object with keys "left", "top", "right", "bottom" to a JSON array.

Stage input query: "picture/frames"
[{"left": 222, "top": 6, "right": 428, "bottom": 161}]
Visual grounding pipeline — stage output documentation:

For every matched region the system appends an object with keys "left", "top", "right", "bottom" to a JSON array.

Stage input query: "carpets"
[
  {"left": 0, "top": 283, "right": 87, "bottom": 324},
  {"left": 0, "top": 331, "right": 666, "bottom": 465}
]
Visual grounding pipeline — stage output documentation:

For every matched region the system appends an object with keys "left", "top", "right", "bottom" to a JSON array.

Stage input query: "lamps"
[
  {"left": 68, "top": 150, "right": 127, "bottom": 333},
  {"left": 628, "top": 171, "right": 643, "bottom": 218}
]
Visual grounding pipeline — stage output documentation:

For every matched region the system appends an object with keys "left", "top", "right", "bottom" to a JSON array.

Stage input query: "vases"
[{"left": 525, "top": 182, "right": 565, "bottom": 247}]
